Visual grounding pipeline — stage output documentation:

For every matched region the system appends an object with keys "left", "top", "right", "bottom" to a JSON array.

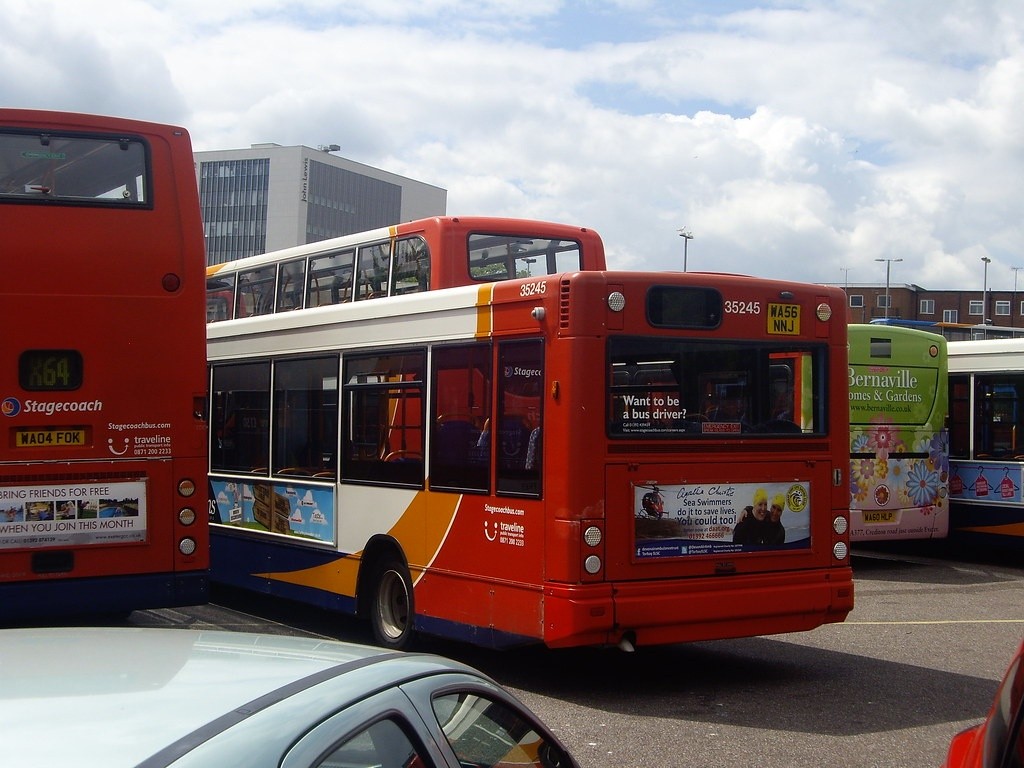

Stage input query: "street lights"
[
  {"left": 676, "top": 225, "right": 693, "bottom": 272},
  {"left": 876, "top": 258, "right": 903, "bottom": 320},
  {"left": 982, "top": 256, "right": 992, "bottom": 324}
]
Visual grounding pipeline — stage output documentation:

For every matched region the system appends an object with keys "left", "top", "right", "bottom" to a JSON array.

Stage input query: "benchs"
[{"left": 615, "top": 363, "right": 792, "bottom": 428}]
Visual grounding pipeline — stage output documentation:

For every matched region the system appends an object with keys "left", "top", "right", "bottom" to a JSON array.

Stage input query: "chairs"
[
  {"left": 433, "top": 408, "right": 543, "bottom": 495},
  {"left": 253, "top": 449, "right": 424, "bottom": 480}
]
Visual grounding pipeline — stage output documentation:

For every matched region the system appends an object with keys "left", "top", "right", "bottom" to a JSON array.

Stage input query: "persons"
[{"left": 733, "top": 488, "right": 787, "bottom": 552}]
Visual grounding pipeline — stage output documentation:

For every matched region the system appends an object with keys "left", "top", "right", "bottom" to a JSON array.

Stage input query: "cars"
[{"left": 0, "top": 623, "right": 582, "bottom": 768}]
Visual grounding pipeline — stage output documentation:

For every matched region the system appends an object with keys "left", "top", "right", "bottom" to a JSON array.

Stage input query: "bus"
[
  {"left": 1, "top": 104, "right": 212, "bottom": 611},
  {"left": 868, "top": 318, "right": 1024, "bottom": 345},
  {"left": 202, "top": 215, "right": 609, "bottom": 469},
  {"left": 617, "top": 324, "right": 952, "bottom": 554},
  {"left": 939, "top": 335, "right": 1024, "bottom": 516},
  {"left": 203, "top": 269, "right": 856, "bottom": 659}
]
